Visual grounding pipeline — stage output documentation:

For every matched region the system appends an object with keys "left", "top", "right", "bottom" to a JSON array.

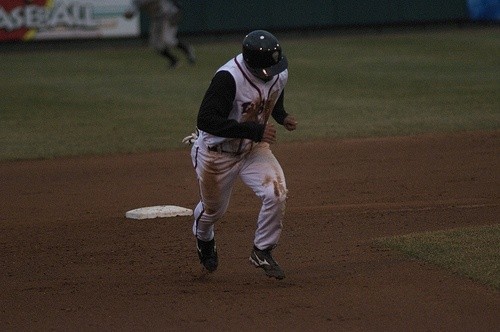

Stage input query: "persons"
[
  {"left": 145, "top": 0, "right": 197, "bottom": 70},
  {"left": 190, "top": 29, "right": 299, "bottom": 280}
]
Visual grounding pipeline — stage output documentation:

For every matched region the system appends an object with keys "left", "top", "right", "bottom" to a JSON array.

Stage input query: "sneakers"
[
  {"left": 195, "top": 237, "right": 220, "bottom": 272},
  {"left": 248, "top": 244, "right": 286, "bottom": 280}
]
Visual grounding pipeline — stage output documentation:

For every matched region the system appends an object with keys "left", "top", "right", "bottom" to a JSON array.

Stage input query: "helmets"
[{"left": 245, "top": 32, "right": 287, "bottom": 77}]
